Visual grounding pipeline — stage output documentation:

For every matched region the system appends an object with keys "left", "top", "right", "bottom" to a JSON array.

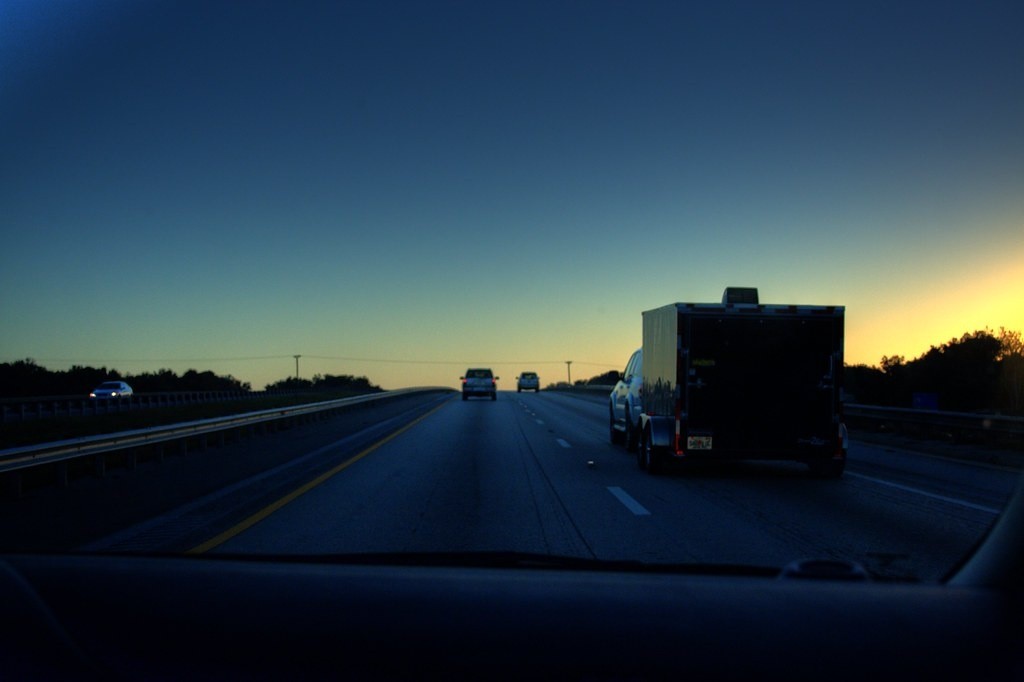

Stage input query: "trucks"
[{"left": 634, "top": 288, "right": 851, "bottom": 480}]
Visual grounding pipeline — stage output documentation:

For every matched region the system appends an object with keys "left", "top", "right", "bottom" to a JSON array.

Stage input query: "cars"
[
  {"left": 515, "top": 371, "right": 541, "bottom": 393},
  {"left": 459, "top": 368, "right": 499, "bottom": 402},
  {"left": 89, "top": 380, "right": 133, "bottom": 398},
  {"left": 610, "top": 349, "right": 642, "bottom": 451}
]
